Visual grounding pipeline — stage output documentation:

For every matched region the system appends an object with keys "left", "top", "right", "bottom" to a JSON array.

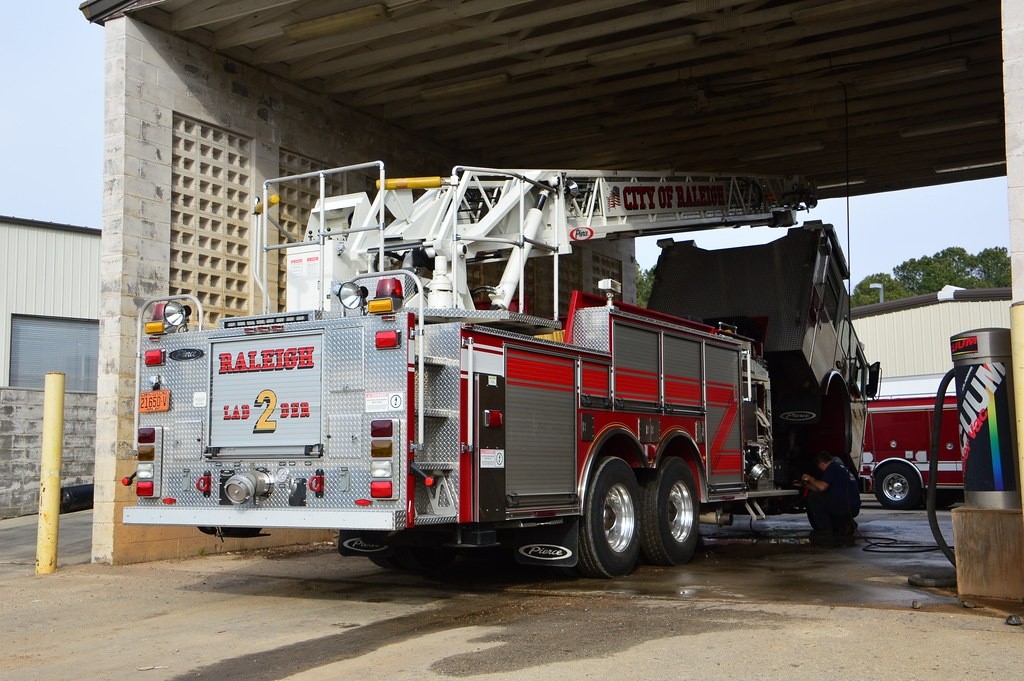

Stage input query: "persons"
[{"left": 790, "top": 450, "right": 863, "bottom": 540}]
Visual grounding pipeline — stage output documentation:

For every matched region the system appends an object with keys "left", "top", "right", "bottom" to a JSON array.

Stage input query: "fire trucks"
[
  {"left": 862, "top": 374, "right": 970, "bottom": 509},
  {"left": 120, "top": 154, "right": 887, "bottom": 578}
]
